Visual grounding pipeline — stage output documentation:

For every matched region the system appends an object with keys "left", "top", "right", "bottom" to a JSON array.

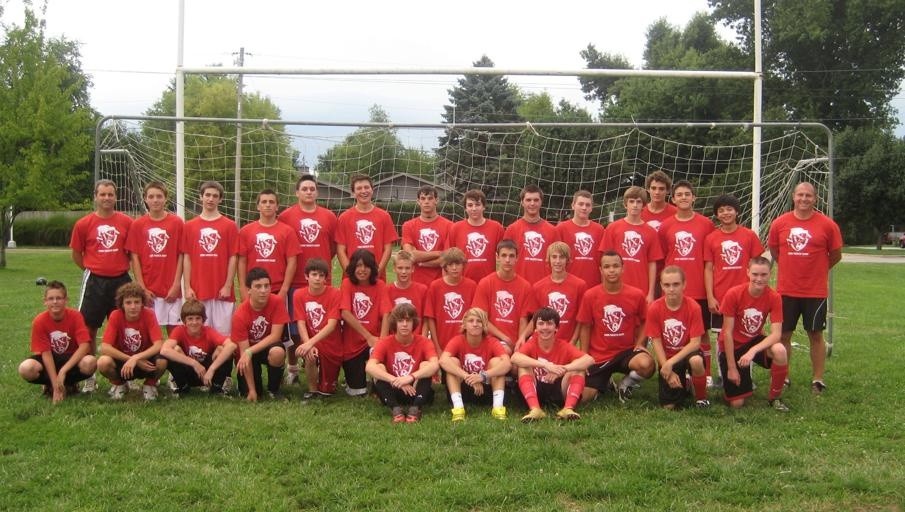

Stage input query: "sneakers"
[
  {"left": 491, "top": 407, "right": 511, "bottom": 421},
  {"left": 522, "top": 408, "right": 548, "bottom": 424},
  {"left": 107, "top": 380, "right": 130, "bottom": 400},
  {"left": 142, "top": 384, "right": 158, "bottom": 400},
  {"left": 450, "top": 408, "right": 467, "bottom": 423},
  {"left": 214, "top": 387, "right": 232, "bottom": 399},
  {"left": 81, "top": 373, "right": 99, "bottom": 394},
  {"left": 200, "top": 383, "right": 214, "bottom": 392},
  {"left": 558, "top": 407, "right": 582, "bottom": 422},
  {"left": 39, "top": 385, "right": 53, "bottom": 399},
  {"left": 606, "top": 374, "right": 827, "bottom": 412},
  {"left": 392, "top": 412, "right": 407, "bottom": 425},
  {"left": 168, "top": 379, "right": 179, "bottom": 392},
  {"left": 238, "top": 391, "right": 248, "bottom": 399},
  {"left": 155, "top": 379, "right": 160, "bottom": 387},
  {"left": 173, "top": 384, "right": 190, "bottom": 399},
  {"left": 221, "top": 376, "right": 233, "bottom": 391},
  {"left": 66, "top": 384, "right": 80, "bottom": 396},
  {"left": 287, "top": 374, "right": 299, "bottom": 387},
  {"left": 268, "top": 391, "right": 288, "bottom": 401},
  {"left": 303, "top": 391, "right": 317, "bottom": 400},
  {"left": 407, "top": 406, "right": 424, "bottom": 423}
]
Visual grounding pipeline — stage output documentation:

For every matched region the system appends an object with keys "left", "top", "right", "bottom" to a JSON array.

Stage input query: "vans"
[{"left": 884, "top": 231, "right": 905, "bottom": 243}]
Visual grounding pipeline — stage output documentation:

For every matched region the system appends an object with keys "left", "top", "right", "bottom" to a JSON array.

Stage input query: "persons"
[
  {"left": 19, "top": 281, "right": 97, "bottom": 398},
  {"left": 770, "top": 183, "right": 845, "bottom": 393},
  {"left": 719, "top": 257, "right": 794, "bottom": 413},
  {"left": 67, "top": 173, "right": 766, "bottom": 424}
]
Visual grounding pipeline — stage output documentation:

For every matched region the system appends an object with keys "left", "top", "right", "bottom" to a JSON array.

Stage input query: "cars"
[{"left": 899, "top": 235, "right": 905, "bottom": 248}]
